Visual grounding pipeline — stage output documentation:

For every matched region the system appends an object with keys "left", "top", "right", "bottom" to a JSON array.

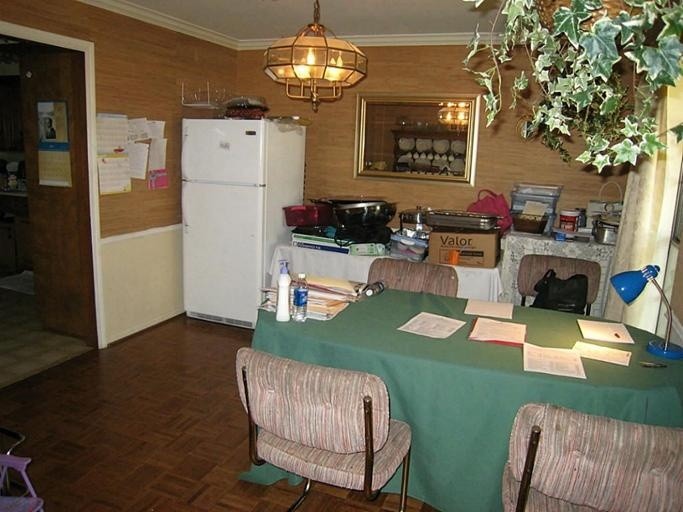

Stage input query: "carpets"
[{"left": 0, "top": 270, "right": 36, "bottom": 296}]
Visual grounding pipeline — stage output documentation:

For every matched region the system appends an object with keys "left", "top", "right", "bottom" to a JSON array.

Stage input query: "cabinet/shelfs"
[
  {"left": 389, "top": 127, "right": 467, "bottom": 177},
  {"left": 0, "top": 74, "right": 25, "bottom": 154},
  {"left": 1, "top": 192, "right": 35, "bottom": 277}
]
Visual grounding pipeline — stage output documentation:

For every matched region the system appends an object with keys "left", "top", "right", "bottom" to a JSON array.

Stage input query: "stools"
[
  {"left": 0, "top": 426, "right": 39, "bottom": 504},
  {"left": 1, "top": 454, "right": 45, "bottom": 512}
]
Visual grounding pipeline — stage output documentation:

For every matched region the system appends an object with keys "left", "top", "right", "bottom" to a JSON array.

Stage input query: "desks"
[
  {"left": 501, "top": 232, "right": 616, "bottom": 322},
  {"left": 274, "top": 241, "right": 512, "bottom": 303},
  {"left": 235, "top": 277, "right": 683, "bottom": 512}
]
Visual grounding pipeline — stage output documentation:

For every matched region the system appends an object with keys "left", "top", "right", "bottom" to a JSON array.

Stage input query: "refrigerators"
[{"left": 181, "top": 118, "right": 308, "bottom": 329}]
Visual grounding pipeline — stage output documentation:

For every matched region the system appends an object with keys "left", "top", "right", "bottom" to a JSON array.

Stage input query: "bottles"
[
  {"left": 365, "top": 281, "right": 386, "bottom": 297},
  {"left": 575, "top": 208, "right": 586, "bottom": 226},
  {"left": 560, "top": 208, "right": 578, "bottom": 233},
  {"left": 292, "top": 274, "right": 308, "bottom": 320}
]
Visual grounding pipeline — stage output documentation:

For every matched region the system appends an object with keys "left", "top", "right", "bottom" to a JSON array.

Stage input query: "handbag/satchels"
[
  {"left": 335, "top": 226, "right": 393, "bottom": 246},
  {"left": 587, "top": 179, "right": 624, "bottom": 216},
  {"left": 465, "top": 188, "right": 512, "bottom": 237},
  {"left": 532, "top": 267, "right": 588, "bottom": 316}
]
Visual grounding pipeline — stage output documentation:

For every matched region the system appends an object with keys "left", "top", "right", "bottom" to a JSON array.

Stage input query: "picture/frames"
[{"left": 35, "top": 99, "right": 69, "bottom": 144}]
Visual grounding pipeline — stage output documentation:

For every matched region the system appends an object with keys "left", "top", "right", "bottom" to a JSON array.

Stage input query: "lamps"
[
  {"left": 436, "top": 103, "right": 473, "bottom": 132},
  {"left": 262, "top": 0, "right": 370, "bottom": 112},
  {"left": 609, "top": 264, "right": 683, "bottom": 359}
]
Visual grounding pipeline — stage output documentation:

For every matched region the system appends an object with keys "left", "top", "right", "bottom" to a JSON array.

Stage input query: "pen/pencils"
[
  {"left": 640, "top": 362, "right": 668, "bottom": 367},
  {"left": 608, "top": 326, "right": 620, "bottom": 338}
]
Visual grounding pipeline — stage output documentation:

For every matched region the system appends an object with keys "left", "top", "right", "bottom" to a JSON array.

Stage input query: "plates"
[{"left": 394, "top": 135, "right": 466, "bottom": 175}]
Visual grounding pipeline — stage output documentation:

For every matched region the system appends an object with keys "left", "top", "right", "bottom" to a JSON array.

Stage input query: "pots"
[{"left": 335, "top": 200, "right": 399, "bottom": 229}]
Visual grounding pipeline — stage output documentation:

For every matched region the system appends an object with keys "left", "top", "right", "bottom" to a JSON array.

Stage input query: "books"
[{"left": 256, "top": 274, "right": 369, "bottom": 321}]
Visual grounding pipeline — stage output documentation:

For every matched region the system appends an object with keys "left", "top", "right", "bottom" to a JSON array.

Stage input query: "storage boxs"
[{"left": 428, "top": 228, "right": 502, "bottom": 268}]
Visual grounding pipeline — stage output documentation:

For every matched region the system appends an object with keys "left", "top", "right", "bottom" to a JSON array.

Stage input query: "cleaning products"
[{"left": 274, "top": 259, "right": 293, "bottom": 322}]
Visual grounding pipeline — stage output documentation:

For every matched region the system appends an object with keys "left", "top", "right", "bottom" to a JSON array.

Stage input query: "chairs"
[
  {"left": 235, "top": 346, "right": 417, "bottom": 512},
  {"left": 498, "top": 398, "right": 683, "bottom": 512},
  {"left": 363, "top": 256, "right": 462, "bottom": 303},
  {"left": 516, "top": 253, "right": 601, "bottom": 317}
]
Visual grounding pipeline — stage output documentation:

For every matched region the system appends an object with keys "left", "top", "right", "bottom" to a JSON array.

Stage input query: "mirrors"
[{"left": 352, "top": 91, "right": 482, "bottom": 188}]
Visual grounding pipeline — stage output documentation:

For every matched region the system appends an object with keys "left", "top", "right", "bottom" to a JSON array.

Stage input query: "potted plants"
[{"left": 455, "top": 0, "right": 683, "bottom": 175}]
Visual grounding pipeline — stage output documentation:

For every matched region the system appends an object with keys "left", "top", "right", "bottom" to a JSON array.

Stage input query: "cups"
[{"left": 543, "top": 213, "right": 556, "bottom": 236}]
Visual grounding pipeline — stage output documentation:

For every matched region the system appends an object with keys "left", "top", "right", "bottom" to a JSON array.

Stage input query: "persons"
[{"left": 44, "top": 118, "right": 56, "bottom": 139}]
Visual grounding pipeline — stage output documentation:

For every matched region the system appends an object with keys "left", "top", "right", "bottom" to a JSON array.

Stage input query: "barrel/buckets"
[{"left": 559, "top": 210, "right": 580, "bottom": 232}]
